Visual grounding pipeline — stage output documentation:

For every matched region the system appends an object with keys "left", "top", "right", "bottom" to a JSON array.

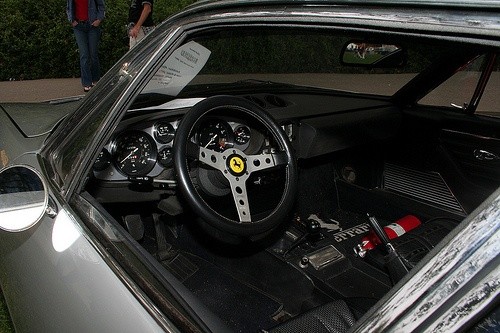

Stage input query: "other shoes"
[
  {"left": 83, "top": 85, "right": 92, "bottom": 92},
  {"left": 91, "top": 81, "right": 97, "bottom": 87}
]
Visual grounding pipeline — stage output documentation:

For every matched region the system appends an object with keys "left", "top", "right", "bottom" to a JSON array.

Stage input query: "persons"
[
  {"left": 128, "top": 0, "right": 155, "bottom": 51},
  {"left": 66, "top": 0, "right": 107, "bottom": 93}
]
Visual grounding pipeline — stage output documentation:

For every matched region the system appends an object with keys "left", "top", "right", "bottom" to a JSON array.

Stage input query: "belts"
[{"left": 76, "top": 20, "right": 87, "bottom": 24}]
